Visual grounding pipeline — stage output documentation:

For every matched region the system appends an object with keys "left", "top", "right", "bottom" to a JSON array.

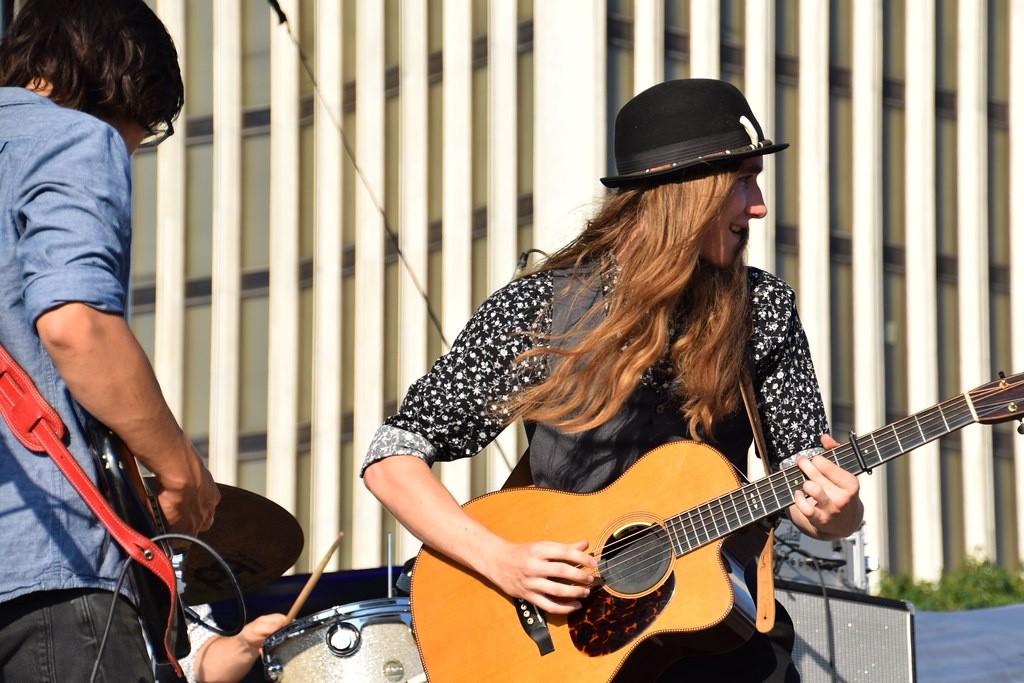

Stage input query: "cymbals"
[{"left": 141, "top": 474, "right": 305, "bottom": 607}]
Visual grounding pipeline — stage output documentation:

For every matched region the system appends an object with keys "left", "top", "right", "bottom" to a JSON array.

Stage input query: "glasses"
[{"left": 138, "top": 116, "right": 175, "bottom": 148}]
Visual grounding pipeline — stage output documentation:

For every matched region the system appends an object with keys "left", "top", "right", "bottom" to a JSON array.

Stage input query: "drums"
[{"left": 261, "top": 595, "right": 426, "bottom": 681}]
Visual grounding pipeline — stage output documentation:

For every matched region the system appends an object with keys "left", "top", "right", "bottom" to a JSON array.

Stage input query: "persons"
[
  {"left": 2, "top": 0, "right": 224, "bottom": 683},
  {"left": 142, "top": 539, "right": 294, "bottom": 683},
  {"left": 357, "top": 73, "right": 866, "bottom": 682}
]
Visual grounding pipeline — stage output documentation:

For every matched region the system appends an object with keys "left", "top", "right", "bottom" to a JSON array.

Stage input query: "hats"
[
  {"left": 598, "top": 79, "right": 790, "bottom": 188},
  {"left": 141, "top": 476, "right": 305, "bottom": 606}
]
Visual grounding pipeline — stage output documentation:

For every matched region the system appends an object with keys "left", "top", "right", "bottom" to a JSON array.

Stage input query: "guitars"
[
  {"left": 408, "top": 370, "right": 1024, "bottom": 682},
  {"left": 84, "top": 412, "right": 192, "bottom": 667}
]
